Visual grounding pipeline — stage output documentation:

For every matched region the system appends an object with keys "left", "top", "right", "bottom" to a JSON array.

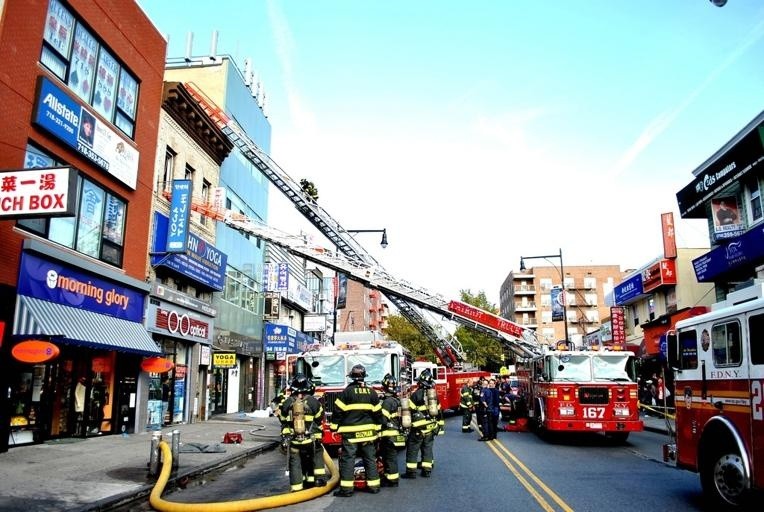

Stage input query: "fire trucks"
[
  {"left": 661, "top": 281, "right": 764, "bottom": 512},
  {"left": 154, "top": 78, "right": 565, "bottom": 423},
  {"left": 158, "top": 192, "right": 643, "bottom": 444}
]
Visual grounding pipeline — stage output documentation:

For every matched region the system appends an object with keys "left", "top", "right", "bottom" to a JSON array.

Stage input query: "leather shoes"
[
  {"left": 401, "top": 470, "right": 432, "bottom": 479},
  {"left": 332, "top": 478, "right": 399, "bottom": 496}
]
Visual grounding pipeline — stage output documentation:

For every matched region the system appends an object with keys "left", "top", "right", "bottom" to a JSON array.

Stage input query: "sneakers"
[{"left": 314, "top": 479, "right": 327, "bottom": 486}]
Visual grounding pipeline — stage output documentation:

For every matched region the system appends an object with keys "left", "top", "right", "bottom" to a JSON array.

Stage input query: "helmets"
[
  {"left": 294, "top": 375, "right": 312, "bottom": 392},
  {"left": 382, "top": 375, "right": 401, "bottom": 393},
  {"left": 420, "top": 369, "right": 435, "bottom": 387},
  {"left": 350, "top": 365, "right": 367, "bottom": 380}
]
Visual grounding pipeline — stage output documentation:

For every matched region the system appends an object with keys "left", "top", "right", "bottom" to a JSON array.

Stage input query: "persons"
[
  {"left": 268, "top": 364, "right": 526, "bottom": 498},
  {"left": 701, "top": 329, "right": 710, "bottom": 351},
  {"left": 299, "top": 178, "right": 318, "bottom": 206},
  {"left": 636, "top": 373, "right": 672, "bottom": 420},
  {"left": 74, "top": 376, "right": 87, "bottom": 413},
  {"left": 161, "top": 375, "right": 171, "bottom": 426},
  {"left": 90, "top": 377, "right": 109, "bottom": 436}
]
